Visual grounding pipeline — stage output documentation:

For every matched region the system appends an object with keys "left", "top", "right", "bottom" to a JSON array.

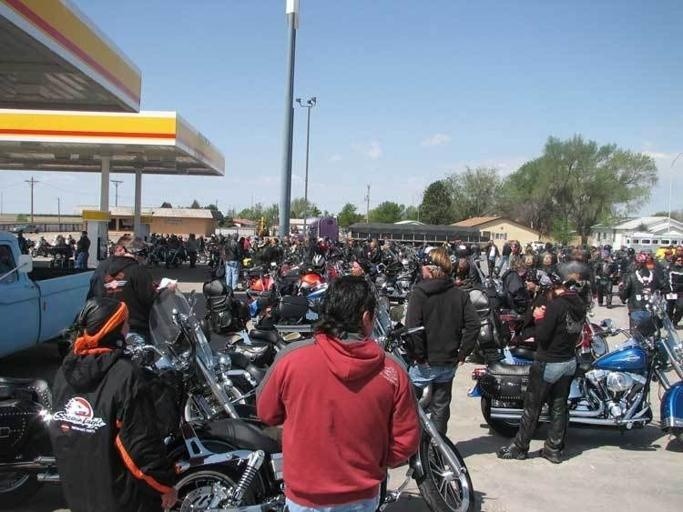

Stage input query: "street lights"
[{"left": 295, "top": 96, "right": 318, "bottom": 235}]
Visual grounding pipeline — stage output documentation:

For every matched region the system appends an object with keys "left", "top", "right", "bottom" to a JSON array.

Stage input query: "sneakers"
[
  {"left": 540, "top": 448, "right": 562, "bottom": 464},
  {"left": 496, "top": 447, "right": 527, "bottom": 460}
]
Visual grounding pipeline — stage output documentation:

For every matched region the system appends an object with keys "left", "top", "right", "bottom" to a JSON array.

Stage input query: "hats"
[{"left": 125, "top": 237, "right": 154, "bottom": 254}]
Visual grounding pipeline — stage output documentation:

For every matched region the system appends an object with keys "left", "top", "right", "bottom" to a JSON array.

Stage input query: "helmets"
[{"left": 312, "top": 255, "right": 326, "bottom": 272}]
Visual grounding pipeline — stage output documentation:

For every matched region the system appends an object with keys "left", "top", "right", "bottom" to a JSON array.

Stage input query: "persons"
[
  {"left": 403, "top": 248, "right": 481, "bottom": 478},
  {"left": 17, "top": 230, "right": 90, "bottom": 268},
  {"left": 50, "top": 297, "right": 178, "bottom": 512},
  {"left": 85, "top": 234, "right": 176, "bottom": 345},
  {"left": 497, "top": 259, "right": 590, "bottom": 464},
  {"left": 254, "top": 274, "right": 421, "bottom": 512},
  {"left": 482, "top": 239, "right": 682, "bottom": 336},
  {"left": 109, "top": 230, "right": 479, "bottom": 290}
]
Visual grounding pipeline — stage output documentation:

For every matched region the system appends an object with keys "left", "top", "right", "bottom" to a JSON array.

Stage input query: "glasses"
[
  {"left": 421, "top": 255, "right": 436, "bottom": 266},
  {"left": 130, "top": 251, "right": 146, "bottom": 257}
]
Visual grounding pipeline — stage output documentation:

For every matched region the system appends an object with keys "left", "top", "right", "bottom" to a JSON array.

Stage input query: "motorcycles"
[
  {"left": 467, "top": 289, "right": 683, "bottom": 445},
  {"left": 0, "top": 227, "right": 610, "bottom": 512}
]
